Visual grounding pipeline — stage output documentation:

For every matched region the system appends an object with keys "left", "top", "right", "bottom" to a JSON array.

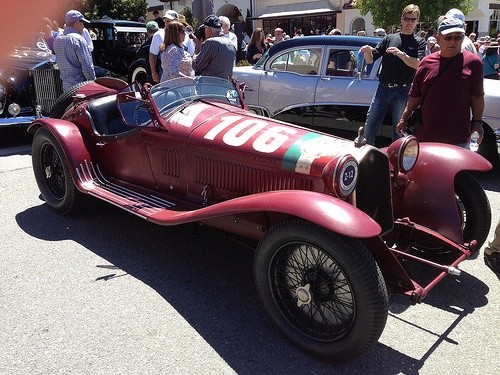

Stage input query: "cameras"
[{"left": 189, "top": 26, "right": 205, "bottom": 39}]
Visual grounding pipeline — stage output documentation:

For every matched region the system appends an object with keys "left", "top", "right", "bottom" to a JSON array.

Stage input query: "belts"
[{"left": 379, "top": 81, "right": 409, "bottom": 88}]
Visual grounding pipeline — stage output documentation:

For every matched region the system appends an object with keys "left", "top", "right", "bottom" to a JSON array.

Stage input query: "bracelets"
[
  {"left": 472, "top": 118, "right": 483, "bottom": 125},
  {"left": 399, "top": 52, "right": 406, "bottom": 59},
  {"left": 152, "top": 72, "right": 156, "bottom": 75}
]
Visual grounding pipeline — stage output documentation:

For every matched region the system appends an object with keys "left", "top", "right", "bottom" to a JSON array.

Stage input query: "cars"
[
  {"left": 233, "top": 35, "right": 500, "bottom": 170},
  {"left": 0, "top": 18, "right": 149, "bottom": 115},
  {"left": 25, "top": 74, "right": 494, "bottom": 362}
]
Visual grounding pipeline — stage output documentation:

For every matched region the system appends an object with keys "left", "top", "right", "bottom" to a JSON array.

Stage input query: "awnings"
[{"left": 148, "top": 5, "right": 164, "bottom": 12}]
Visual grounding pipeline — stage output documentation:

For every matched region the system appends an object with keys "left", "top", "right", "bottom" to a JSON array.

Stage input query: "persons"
[
  {"left": 396, "top": 18, "right": 484, "bottom": 195},
  {"left": 361, "top": 4, "right": 427, "bottom": 149},
  {"left": 37, "top": 10, "right": 239, "bottom": 85},
  {"left": 242, "top": 8, "right": 500, "bottom": 80},
  {"left": 53, "top": 10, "right": 96, "bottom": 111}
]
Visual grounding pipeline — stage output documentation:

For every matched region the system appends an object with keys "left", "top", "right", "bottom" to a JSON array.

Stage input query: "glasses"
[
  {"left": 404, "top": 17, "right": 417, "bottom": 23},
  {"left": 442, "top": 35, "right": 463, "bottom": 41}
]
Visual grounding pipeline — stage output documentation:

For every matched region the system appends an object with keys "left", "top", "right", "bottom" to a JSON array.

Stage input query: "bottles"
[{"left": 469, "top": 129, "right": 480, "bottom": 152}]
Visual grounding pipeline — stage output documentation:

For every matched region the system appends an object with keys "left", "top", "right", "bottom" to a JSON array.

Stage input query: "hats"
[
  {"left": 203, "top": 14, "right": 222, "bottom": 29},
  {"left": 428, "top": 36, "right": 436, "bottom": 44},
  {"left": 146, "top": 21, "right": 158, "bottom": 31},
  {"left": 438, "top": 19, "right": 465, "bottom": 35},
  {"left": 161, "top": 10, "right": 179, "bottom": 21},
  {"left": 445, "top": 8, "right": 465, "bottom": 21},
  {"left": 64, "top": 9, "right": 91, "bottom": 25},
  {"left": 177, "top": 14, "right": 189, "bottom": 26}
]
